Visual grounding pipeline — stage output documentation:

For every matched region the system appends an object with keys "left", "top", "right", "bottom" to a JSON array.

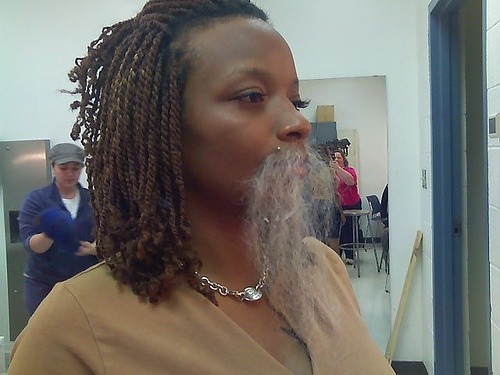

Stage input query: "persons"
[
  {"left": 304, "top": 144, "right": 346, "bottom": 261},
  {"left": 0, "top": 0, "right": 399, "bottom": 375},
  {"left": 380, "top": 184, "right": 388, "bottom": 253},
  {"left": 18, "top": 144, "right": 101, "bottom": 326},
  {"left": 331, "top": 151, "right": 362, "bottom": 265}
]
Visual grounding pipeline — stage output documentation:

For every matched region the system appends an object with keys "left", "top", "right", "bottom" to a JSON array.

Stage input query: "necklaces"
[{"left": 171, "top": 249, "right": 280, "bottom": 302}]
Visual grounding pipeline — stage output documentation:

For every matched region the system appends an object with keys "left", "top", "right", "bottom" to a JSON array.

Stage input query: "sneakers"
[{"left": 346, "top": 258, "right": 354, "bottom": 265}]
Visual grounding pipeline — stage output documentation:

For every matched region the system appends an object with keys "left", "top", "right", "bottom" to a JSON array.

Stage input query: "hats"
[{"left": 47, "top": 143, "right": 84, "bottom": 167}]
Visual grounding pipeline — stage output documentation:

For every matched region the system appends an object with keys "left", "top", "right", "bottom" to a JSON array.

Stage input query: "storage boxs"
[{"left": 317, "top": 105, "right": 334, "bottom": 124}]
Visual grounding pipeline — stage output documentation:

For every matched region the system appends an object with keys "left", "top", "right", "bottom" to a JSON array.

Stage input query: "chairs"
[{"left": 364, "top": 195, "right": 382, "bottom": 252}]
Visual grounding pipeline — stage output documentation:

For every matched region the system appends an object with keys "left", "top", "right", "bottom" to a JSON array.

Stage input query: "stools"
[{"left": 339, "top": 209, "right": 380, "bottom": 279}]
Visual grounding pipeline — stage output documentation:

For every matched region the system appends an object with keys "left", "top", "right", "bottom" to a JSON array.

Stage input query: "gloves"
[{"left": 40, "top": 209, "right": 73, "bottom": 241}]
